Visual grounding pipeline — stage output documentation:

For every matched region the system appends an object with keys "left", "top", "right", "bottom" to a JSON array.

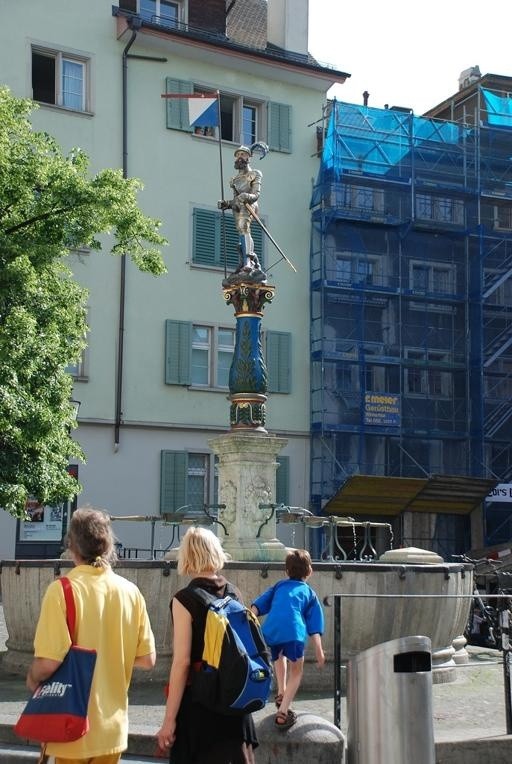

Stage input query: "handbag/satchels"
[{"left": 13, "top": 643, "right": 97, "bottom": 742}]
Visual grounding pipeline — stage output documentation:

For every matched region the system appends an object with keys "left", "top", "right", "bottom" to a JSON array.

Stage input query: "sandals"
[
  {"left": 274, "top": 695, "right": 284, "bottom": 707},
  {"left": 274, "top": 709, "right": 297, "bottom": 730}
]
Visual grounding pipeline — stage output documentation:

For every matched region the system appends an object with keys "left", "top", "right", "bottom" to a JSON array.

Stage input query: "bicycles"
[{"left": 445, "top": 546, "right": 512, "bottom": 651}]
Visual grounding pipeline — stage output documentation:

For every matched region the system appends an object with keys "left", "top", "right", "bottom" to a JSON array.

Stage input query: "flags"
[{"left": 161, "top": 91, "right": 219, "bottom": 128}]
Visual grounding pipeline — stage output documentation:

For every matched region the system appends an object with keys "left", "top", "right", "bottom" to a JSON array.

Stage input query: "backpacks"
[{"left": 184, "top": 581, "right": 274, "bottom": 718}]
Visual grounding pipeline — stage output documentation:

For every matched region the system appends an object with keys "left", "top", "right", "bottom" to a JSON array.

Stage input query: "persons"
[
  {"left": 25, "top": 506, "right": 158, "bottom": 763},
  {"left": 154, "top": 525, "right": 260, "bottom": 764},
  {"left": 247, "top": 548, "right": 327, "bottom": 730},
  {"left": 216, "top": 140, "right": 271, "bottom": 271},
  {"left": 464, "top": 608, "right": 487, "bottom": 645}
]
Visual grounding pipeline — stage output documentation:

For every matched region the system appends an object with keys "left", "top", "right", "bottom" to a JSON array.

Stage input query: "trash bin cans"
[{"left": 345, "top": 635, "right": 436, "bottom": 764}]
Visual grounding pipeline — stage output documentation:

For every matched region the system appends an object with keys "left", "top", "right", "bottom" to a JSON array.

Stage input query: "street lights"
[{"left": 57, "top": 392, "right": 82, "bottom": 557}]
